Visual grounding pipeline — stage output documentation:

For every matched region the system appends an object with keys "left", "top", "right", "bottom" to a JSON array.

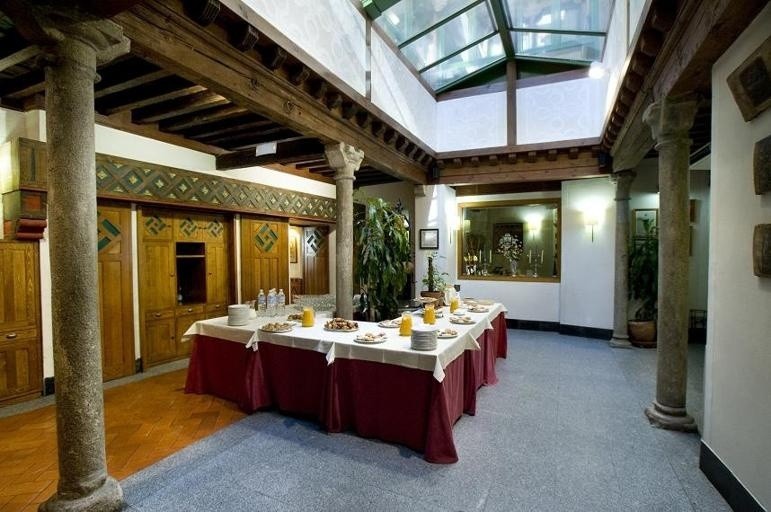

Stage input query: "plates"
[
  {"left": 324, "top": 318, "right": 358, "bottom": 332},
  {"left": 259, "top": 323, "right": 295, "bottom": 333},
  {"left": 468, "top": 305, "right": 489, "bottom": 312},
  {"left": 227, "top": 304, "right": 248, "bottom": 325},
  {"left": 378, "top": 320, "right": 399, "bottom": 328},
  {"left": 287, "top": 314, "right": 302, "bottom": 322},
  {"left": 354, "top": 333, "right": 384, "bottom": 343},
  {"left": 449, "top": 316, "right": 474, "bottom": 324},
  {"left": 411, "top": 326, "right": 437, "bottom": 350},
  {"left": 435, "top": 328, "right": 458, "bottom": 338}
]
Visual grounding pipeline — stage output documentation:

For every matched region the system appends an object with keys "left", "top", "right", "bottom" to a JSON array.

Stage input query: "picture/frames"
[{"left": 419, "top": 228, "right": 439, "bottom": 250}]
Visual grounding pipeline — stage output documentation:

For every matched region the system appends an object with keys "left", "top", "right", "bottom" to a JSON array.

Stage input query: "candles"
[
  {"left": 479, "top": 249, "right": 492, "bottom": 263},
  {"left": 529, "top": 249, "right": 543, "bottom": 264}
]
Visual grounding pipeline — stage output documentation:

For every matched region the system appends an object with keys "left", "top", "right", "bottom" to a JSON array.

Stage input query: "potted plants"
[
  {"left": 621, "top": 218, "right": 659, "bottom": 349},
  {"left": 420, "top": 252, "right": 449, "bottom": 307}
]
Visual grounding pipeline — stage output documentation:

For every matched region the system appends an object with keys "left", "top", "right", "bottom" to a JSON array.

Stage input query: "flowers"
[{"left": 498, "top": 235, "right": 525, "bottom": 261}]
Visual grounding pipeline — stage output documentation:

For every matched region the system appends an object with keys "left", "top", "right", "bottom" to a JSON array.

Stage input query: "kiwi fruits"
[{"left": 288, "top": 314, "right": 301, "bottom": 320}]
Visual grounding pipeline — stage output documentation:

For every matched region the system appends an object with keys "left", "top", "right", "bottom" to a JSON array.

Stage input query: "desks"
[{"left": 180, "top": 293, "right": 508, "bottom": 464}]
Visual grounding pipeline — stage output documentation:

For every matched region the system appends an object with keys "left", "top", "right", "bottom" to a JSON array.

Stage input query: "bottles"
[
  {"left": 444, "top": 285, "right": 456, "bottom": 306},
  {"left": 257, "top": 287, "right": 285, "bottom": 317}
]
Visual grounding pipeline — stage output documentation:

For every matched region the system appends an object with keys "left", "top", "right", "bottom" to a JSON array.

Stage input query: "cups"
[
  {"left": 301, "top": 305, "right": 313, "bottom": 326},
  {"left": 400, "top": 311, "right": 412, "bottom": 336},
  {"left": 450, "top": 297, "right": 458, "bottom": 313},
  {"left": 423, "top": 303, "right": 435, "bottom": 324}
]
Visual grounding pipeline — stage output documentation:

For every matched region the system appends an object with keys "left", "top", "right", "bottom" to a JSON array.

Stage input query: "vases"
[{"left": 503, "top": 259, "right": 518, "bottom": 275}]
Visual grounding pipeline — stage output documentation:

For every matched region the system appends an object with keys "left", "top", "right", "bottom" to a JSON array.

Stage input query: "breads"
[
  {"left": 357, "top": 331, "right": 386, "bottom": 342},
  {"left": 263, "top": 322, "right": 290, "bottom": 332},
  {"left": 326, "top": 317, "right": 357, "bottom": 331}
]
